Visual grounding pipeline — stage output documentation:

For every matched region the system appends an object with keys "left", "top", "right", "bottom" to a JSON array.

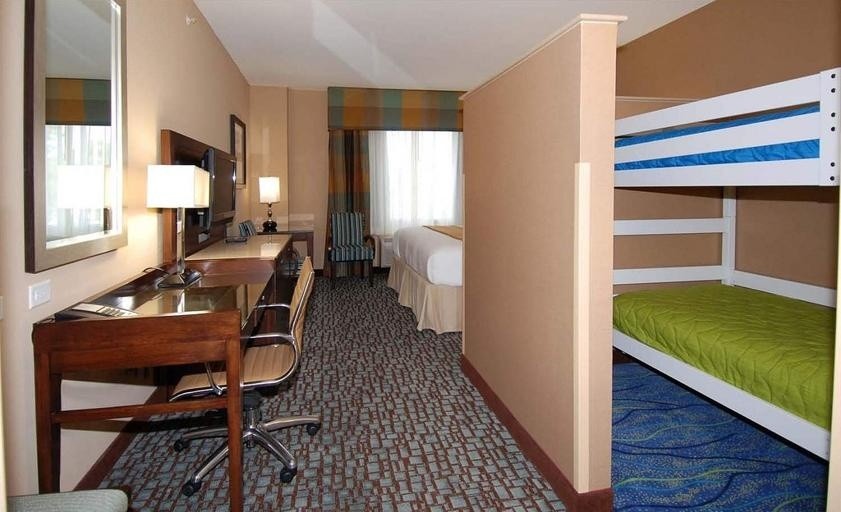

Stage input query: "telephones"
[{"left": 51, "top": 299, "right": 139, "bottom": 320}]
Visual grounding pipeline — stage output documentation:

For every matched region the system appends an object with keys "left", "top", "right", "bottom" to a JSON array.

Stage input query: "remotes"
[{"left": 226, "top": 238, "right": 247, "bottom": 243}]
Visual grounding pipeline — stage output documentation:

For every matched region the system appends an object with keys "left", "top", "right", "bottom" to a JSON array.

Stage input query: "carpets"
[{"left": 612, "top": 361, "right": 829, "bottom": 512}]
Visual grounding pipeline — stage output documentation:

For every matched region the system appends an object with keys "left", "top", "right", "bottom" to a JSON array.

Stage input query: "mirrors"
[{"left": 21, "top": 0, "right": 131, "bottom": 277}]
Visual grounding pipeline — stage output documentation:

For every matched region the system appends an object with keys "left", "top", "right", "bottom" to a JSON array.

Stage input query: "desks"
[{"left": 32, "top": 230, "right": 299, "bottom": 512}]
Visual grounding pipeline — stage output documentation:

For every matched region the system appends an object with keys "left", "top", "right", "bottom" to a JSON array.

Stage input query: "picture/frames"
[{"left": 228, "top": 112, "right": 248, "bottom": 191}]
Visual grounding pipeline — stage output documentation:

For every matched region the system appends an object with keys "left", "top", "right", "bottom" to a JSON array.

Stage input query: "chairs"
[
  {"left": 326, "top": 210, "right": 376, "bottom": 292},
  {"left": 169, "top": 254, "right": 324, "bottom": 497}
]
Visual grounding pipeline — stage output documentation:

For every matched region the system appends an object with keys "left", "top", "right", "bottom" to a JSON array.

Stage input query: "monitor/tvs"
[{"left": 199, "top": 147, "right": 237, "bottom": 230}]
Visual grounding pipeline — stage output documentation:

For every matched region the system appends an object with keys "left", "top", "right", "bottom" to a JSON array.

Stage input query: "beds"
[
  {"left": 615, "top": 66, "right": 840, "bottom": 467},
  {"left": 389, "top": 225, "right": 465, "bottom": 336}
]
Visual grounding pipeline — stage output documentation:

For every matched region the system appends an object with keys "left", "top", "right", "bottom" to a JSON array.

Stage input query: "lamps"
[
  {"left": 145, "top": 162, "right": 214, "bottom": 293},
  {"left": 258, "top": 176, "right": 283, "bottom": 234}
]
